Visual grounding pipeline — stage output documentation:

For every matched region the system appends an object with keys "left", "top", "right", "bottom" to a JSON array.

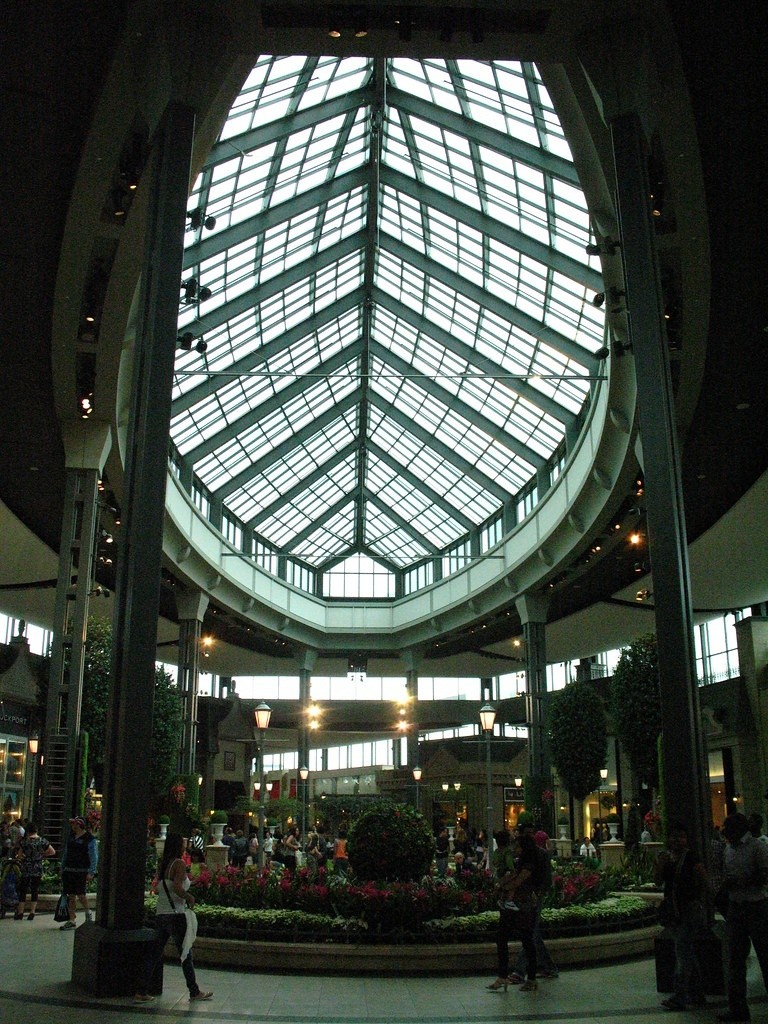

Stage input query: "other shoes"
[
  {"left": 535, "top": 971, "right": 558, "bottom": 980},
  {"left": 60, "top": 920, "right": 77, "bottom": 931},
  {"left": 507, "top": 974, "right": 525, "bottom": 984},
  {"left": 714, "top": 1006, "right": 751, "bottom": 1022},
  {"left": 661, "top": 995, "right": 692, "bottom": 1010},
  {"left": 13, "top": 912, "right": 23, "bottom": 919},
  {"left": 86, "top": 911, "right": 93, "bottom": 921},
  {"left": 133, "top": 994, "right": 158, "bottom": 1003},
  {"left": 498, "top": 899, "right": 519, "bottom": 911},
  {"left": 26, "top": 913, "right": 34, "bottom": 921},
  {"left": 189, "top": 990, "right": 214, "bottom": 1001}
]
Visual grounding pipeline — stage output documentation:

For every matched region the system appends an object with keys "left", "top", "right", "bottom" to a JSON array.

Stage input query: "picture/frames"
[{"left": 225, "top": 751, "right": 236, "bottom": 770}]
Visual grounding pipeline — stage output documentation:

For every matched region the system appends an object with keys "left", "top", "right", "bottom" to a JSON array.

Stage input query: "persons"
[
  {"left": 0, "top": 819, "right": 30, "bottom": 857},
  {"left": 221, "top": 825, "right": 348, "bottom": 870},
  {"left": 715, "top": 813, "right": 768, "bottom": 1024},
  {"left": 436, "top": 823, "right": 489, "bottom": 872},
  {"left": 186, "top": 828, "right": 208, "bottom": 849},
  {"left": 13, "top": 823, "right": 57, "bottom": 920},
  {"left": 187, "top": 850, "right": 206, "bottom": 875},
  {"left": 58, "top": 815, "right": 99, "bottom": 930},
  {"left": 579, "top": 837, "right": 597, "bottom": 858},
  {"left": 655, "top": 822, "right": 721, "bottom": 1007},
  {"left": 133, "top": 834, "right": 214, "bottom": 1003},
  {"left": 483, "top": 824, "right": 558, "bottom": 993}
]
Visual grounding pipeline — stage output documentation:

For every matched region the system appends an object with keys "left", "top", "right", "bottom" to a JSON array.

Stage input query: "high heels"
[
  {"left": 485, "top": 978, "right": 509, "bottom": 993},
  {"left": 519, "top": 976, "right": 536, "bottom": 992}
]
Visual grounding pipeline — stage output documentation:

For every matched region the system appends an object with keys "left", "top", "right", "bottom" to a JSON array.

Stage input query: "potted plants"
[
  {"left": 606, "top": 813, "right": 619, "bottom": 843},
  {"left": 447, "top": 819, "right": 456, "bottom": 839},
  {"left": 558, "top": 817, "right": 569, "bottom": 840},
  {"left": 209, "top": 810, "right": 229, "bottom": 846},
  {"left": 159, "top": 814, "right": 170, "bottom": 838}
]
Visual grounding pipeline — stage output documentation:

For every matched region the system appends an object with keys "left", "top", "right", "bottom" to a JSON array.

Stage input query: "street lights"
[
  {"left": 298, "top": 764, "right": 309, "bottom": 852},
  {"left": 412, "top": 764, "right": 423, "bottom": 811},
  {"left": 28, "top": 733, "right": 40, "bottom": 823},
  {"left": 253, "top": 699, "right": 274, "bottom": 869},
  {"left": 479, "top": 702, "right": 497, "bottom": 870}
]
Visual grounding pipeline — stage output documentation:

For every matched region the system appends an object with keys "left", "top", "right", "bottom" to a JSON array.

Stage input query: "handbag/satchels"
[
  {"left": 54, "top": 891, "right": 77, "bottom": 922},
  {"left": 175, "top": 912, "right": 187, "bottom": 935},
  {"left": 654, "top": 931, "right": 676, "bottom": 994}
]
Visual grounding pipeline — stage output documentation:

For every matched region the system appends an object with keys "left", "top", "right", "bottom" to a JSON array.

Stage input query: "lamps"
[
  {"left": 186, "top": 208, "right": 216, "bottom": 233},
  {"left": 594, "top": 339, "right": 632, "bottom": 361},
  {"left": 180, "top": 277, "right": 211, "bottom": 305},
  {"left": 586, "top": 236, "right": 619, "bottom": 256},
  {"left": 593, "top": 287, "right": 627, "bottom": 310},
  {"left": 647, "top": 180, "right": 688, "bottom": 351},
  {"left": 29, "top": 730, "right": 39, "bottom": 754},
  {"left": 327, "top": 18, "right": 485, "bottom": 42},
  {"left": 82, "top": 178, "right": 138, "bottom": 597},
  {"left": 637, "top": 589, "right": 653, "bottom": 602},
  {"left": 177, "top": 331, "right": 209, "bottom": 353}
]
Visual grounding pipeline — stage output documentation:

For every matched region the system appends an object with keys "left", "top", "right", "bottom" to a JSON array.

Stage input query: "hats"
[{"left": 69, "top": 815, "right": 85, "bottom": 826}]
